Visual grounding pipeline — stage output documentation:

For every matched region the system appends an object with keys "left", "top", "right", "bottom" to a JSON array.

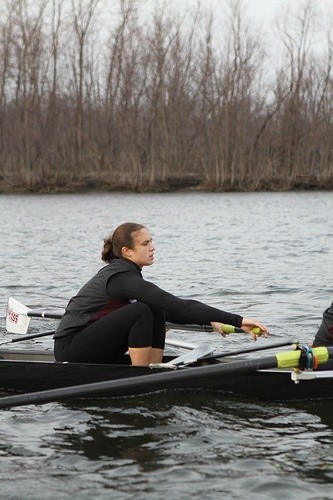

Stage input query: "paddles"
[
  {"left": 0, "top": 345, "right": 333, "bottom": 411},
  {"left": 3, "top": 295, "right": 266, "bottom": 339}
]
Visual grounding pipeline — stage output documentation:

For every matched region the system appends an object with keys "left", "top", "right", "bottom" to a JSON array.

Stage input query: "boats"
[{"left": 0, "top": 347, "right": 333, "bottom": 403}]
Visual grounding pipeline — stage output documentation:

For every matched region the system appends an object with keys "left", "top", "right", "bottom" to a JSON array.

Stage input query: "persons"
[
  {"left": 311, "top": 301, "right": 333, "bottom": 371},
  {"left": 52, "top": 222, "right": 269, "bottom": 366}
]
